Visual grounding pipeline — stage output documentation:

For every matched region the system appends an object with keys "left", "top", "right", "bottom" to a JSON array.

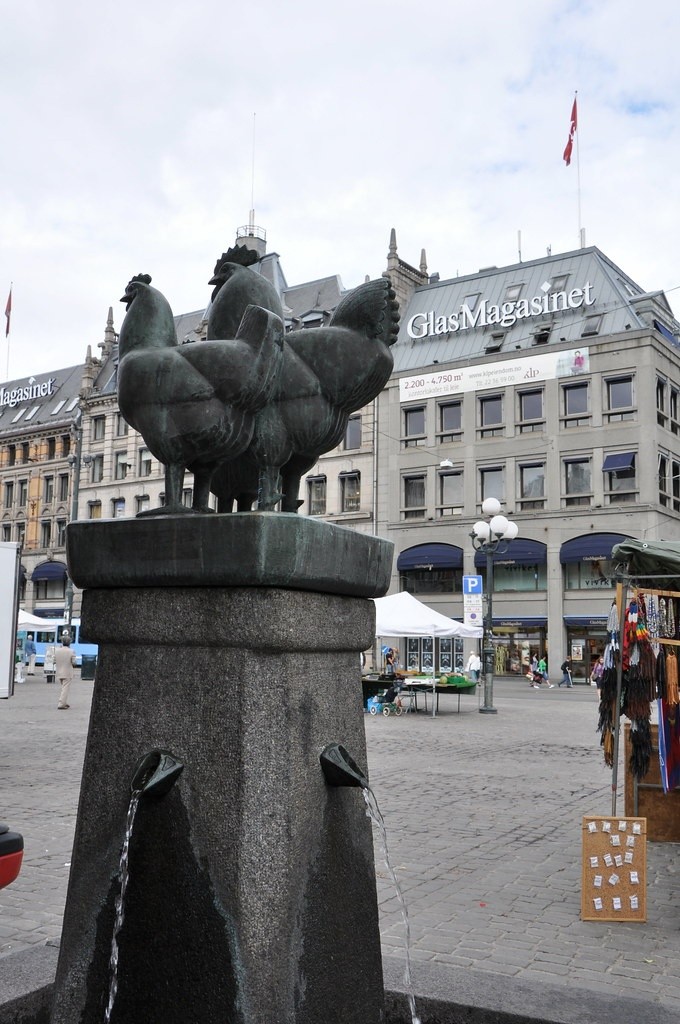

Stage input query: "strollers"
[{"left": 366, "top": 689, "right": 403, "bottom": 718}]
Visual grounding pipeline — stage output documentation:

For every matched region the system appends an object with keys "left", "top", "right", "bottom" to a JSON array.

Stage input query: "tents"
[
  {"left": 17, "top": 608, "right": 57, "bottom": 684},
  {"left": 373, "top": 591, "right": 483, "bottom": 719}
]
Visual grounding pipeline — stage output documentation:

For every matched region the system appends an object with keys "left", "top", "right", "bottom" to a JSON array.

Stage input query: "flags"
[
  {"left": 562, "top": 99, "right": 577, "bottom": 166},
  {"left": 4, "top": 290, "right": 11, "bottom": 337}
]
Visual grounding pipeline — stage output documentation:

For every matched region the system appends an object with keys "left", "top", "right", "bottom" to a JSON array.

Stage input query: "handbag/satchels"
[
  {"left": 527, "top": 670, "right": 533, "bottom": 675},
  {"left": 592, "top": 673, "right": 599, "bottom": 681},
  {"left": 533, "top": 671, "right": 540, "bottom": 677},
  {"left": 533, "top": 673, "right": 543, "bottom": 684}
]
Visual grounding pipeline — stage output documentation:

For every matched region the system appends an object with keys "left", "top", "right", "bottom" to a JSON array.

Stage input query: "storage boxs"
[{"left": 367, "top": 697, "right": 384, "bottom": 713}]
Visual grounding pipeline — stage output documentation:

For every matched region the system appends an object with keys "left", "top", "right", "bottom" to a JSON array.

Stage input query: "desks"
[{"left": 361, "top": 678, "right": 477, "bottom": 714}]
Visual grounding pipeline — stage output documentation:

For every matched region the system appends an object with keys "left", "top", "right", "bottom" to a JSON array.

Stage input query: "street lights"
[{"left": 470, "top": 498, "right": 519, "bottom": 714}]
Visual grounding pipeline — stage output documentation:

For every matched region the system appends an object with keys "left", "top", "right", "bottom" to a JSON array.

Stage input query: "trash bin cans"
[{"left": 81, "top": 654, "right": 97, "bottom": 681}]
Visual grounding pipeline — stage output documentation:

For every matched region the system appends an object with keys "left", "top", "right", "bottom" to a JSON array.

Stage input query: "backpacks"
[{"left": 561, "top": 662, "right": 569, "bottom": 670}]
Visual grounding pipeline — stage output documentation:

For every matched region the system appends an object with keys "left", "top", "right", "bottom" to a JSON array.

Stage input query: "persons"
[
  {"left": 385, "top": 648, "right": 399, "bottom": 673},
  {"left": 468, "top": 651, "right": 483, "bottom": 682},
  {"left": 24, "top": 634, "right": 36, "bottom": 676},
  {"left": 529, "top": 652, "right": 605, "bottom": 703},
  {"left": 53, "top": 636, "right": 76, "bottom": 710}
]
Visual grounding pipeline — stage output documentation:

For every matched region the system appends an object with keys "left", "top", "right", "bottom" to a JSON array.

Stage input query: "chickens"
[{"left": 116, "top": 243, "right": 401, "bottom": 518}]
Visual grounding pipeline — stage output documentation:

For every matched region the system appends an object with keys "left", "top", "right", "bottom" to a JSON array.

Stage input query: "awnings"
[
  {"left": 34, "top": 607, "right": 64, "bottom": 616},
  {"left": 451, "top": 615, "right": 609, "bottom": 628},
  {"left": 473, "top": 538, "right": 547, "bottom": 569},
  {"left": 602, "top": 453, "right": 636, "bottom": 472},
  {"left": 20, "top": 565, "right": 27, "bottom": 583},
  {"left": 559, "top": 533, "right": 636, "bottom": 561},
  {"left": 31, "top": 562, "right": 68, "bottom": 582},
  {"left": 397, "top": 542, "right": 463, "bottom": 572}
]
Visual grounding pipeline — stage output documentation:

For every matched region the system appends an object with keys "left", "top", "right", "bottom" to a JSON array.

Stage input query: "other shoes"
[
  {"left": 58, "top": 707, "right": 68, "bottom": 709},
  {"left": 558, "top": 683, "right": 560, "bottom": 687},
  {"left": 534, "top": 686, "right": 539, "bottom": 689},
  {"left": 65, "top": 705, "right": 69, "bottom": 707},
  {"left": 530, "top": 683, "right": 533, "bottom": 686},
  {"left": 549, "top": 685, "right": 554, "bottom": 688},
  {"left": 31, "top": 673, "right": 34, "bottom": 675},
  {"left": 27, "top": 673, "right": 31, "bottom": 675},
  {"left": 567, "top": 686, "right": 571, "bottom": 688}
]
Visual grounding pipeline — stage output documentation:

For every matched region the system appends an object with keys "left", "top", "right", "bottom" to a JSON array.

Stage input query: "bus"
[{"left": 13, "top": 620, "right": 99, "bottom": 667}]
